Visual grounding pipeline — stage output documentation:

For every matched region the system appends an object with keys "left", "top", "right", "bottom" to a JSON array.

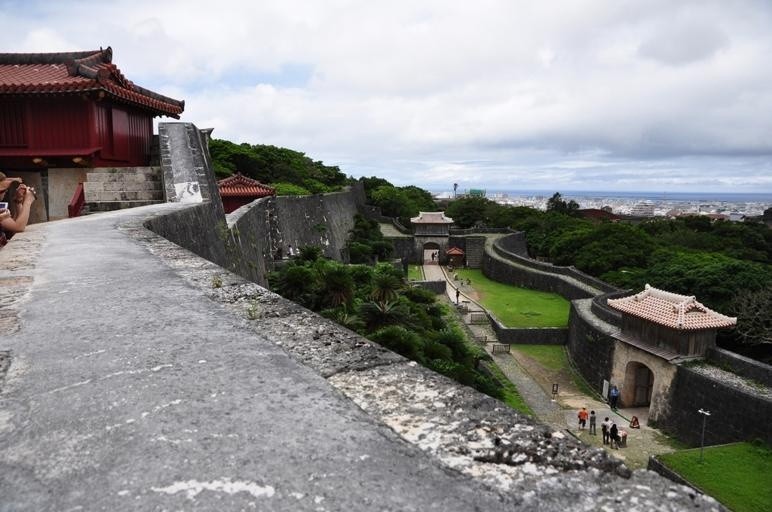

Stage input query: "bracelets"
[{"left": 15, "top": 199, "right": 23, "bottom": 203}]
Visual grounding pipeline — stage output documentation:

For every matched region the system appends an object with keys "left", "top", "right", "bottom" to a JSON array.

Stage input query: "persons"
[
  {"left": 600, "top": 416, "right": 612, "bottom": 445},
  {"left": 431, "top": 253, "right": 434, "bottom": 260},
  {"left": 455, "top": 288, "right": 460, "bottom": 304},
  {"left": 609, "top": 423, "right": 619, "bottom": 450},
  {"left": 610, "top": 385, "right": 620, "bottom": 410},
  {"left": 588, "top": 410, "right": 597, "bottom": 436},
  {"left": 577, "top": 407, "right": 588, "bottom": 429},
  {"left": 0, "top": 171, "right": 38, "bottom": 250}
]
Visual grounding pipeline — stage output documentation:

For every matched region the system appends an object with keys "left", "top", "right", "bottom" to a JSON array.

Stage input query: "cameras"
[{"left": 0, "top": 202, "right": 8, "bottom": 211}]
